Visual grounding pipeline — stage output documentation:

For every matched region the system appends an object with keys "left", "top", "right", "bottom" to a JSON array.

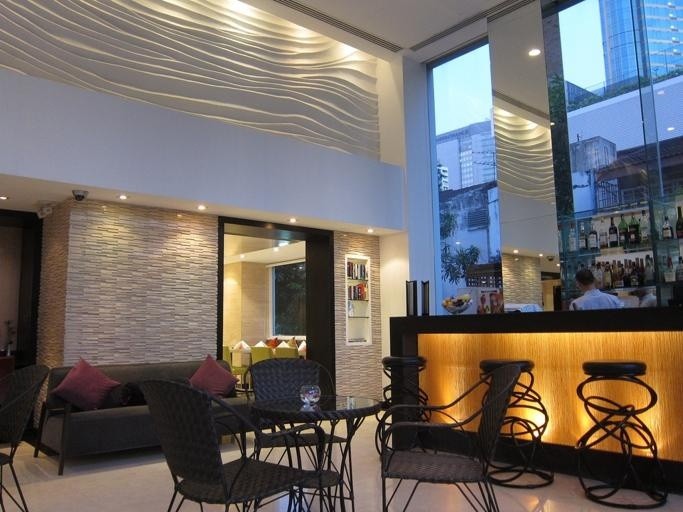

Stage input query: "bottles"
[
  {"left": 567, "top": 204, "right": 682, "bottom": 252},
  {"left": 566, "top": 253, "right": 683, "bottom": 291},
  {"left": 347, "top": 301, "right": 354, "bottom": 316}
]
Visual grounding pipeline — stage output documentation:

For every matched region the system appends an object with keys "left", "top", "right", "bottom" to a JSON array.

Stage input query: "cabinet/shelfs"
[
  {"left": 0, "top": 353, "right": 15, "bottom": 376},
  {"left": 647, "top": 195, "right": 682, "bottom": 308},
  {"left": 343, "top": 253, "right": 372, "bottom": 348},
  {"left": 556, "top": 199, "right": 665, "bottom": 308}
]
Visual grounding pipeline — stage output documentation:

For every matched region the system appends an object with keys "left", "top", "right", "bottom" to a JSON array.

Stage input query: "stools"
[
  {"left": 475, "top": 353, "right": 556, "bottom": 491},
  {"left": 372, "top": 353, "right": 440, "bottom": 464},
  {"left": 571, "top": 357, "right": 670, "bottom": 510}
]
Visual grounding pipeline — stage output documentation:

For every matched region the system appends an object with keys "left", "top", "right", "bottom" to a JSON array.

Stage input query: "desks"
[{"left": 242, "top": 389, "right": 383, "bottom": 512}]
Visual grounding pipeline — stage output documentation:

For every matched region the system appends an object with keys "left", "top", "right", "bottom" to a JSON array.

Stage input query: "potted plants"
[{"left": 1, "top": 318, "right": 15, "bottom": 357}]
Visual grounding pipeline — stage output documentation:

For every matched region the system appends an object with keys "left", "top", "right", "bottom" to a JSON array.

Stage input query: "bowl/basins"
[{"left": 441, "top": 301, "right": 472, "bottom": 314}]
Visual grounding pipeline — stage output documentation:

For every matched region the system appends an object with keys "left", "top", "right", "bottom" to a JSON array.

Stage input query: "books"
[{"left": 348, "top": 262, "right": 368, "bottom": 300}]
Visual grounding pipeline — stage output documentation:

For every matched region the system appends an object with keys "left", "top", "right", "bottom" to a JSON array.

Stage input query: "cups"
[{"left": 299, "top": 384, "right": 320, "bottom": 404}]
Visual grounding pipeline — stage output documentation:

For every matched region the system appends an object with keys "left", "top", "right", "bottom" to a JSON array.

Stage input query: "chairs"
[
  {"left": 1, "top": 363, "right": 48, "bottom": 512},
  {"left": 373, "top": 360, "right": 523, "bottom": 512},
  {"left": 239, "top": 353, "right": 343, "bottom": 511},
  {"left": 218, "top": 334, "right": 307, "bottom": 391},
  {"left": 131, "top": 375, "right": 333, "bottom": 511}
]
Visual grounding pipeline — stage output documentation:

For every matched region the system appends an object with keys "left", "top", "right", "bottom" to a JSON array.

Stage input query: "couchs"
[{"left": 27, "top": 356, "right": 279, "bottom": 477}]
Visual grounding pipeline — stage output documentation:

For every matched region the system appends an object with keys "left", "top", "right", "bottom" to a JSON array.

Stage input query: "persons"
[
  {"left": 570, "top": 269, "right": 624, "bottom": 311},
  {"left": 633, "top": 287, "right": 658, "bottom": 308}
]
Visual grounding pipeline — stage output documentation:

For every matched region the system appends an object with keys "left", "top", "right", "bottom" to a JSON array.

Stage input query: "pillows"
[
  {"left": 120, "top": 379, "right": 144, "bottom": 404},
  {"left": 47, "top": 354, "right": 123, "bottom": 412},
  {"left": 186, "top": 352, "right": 240, "bottom": 400}
]
